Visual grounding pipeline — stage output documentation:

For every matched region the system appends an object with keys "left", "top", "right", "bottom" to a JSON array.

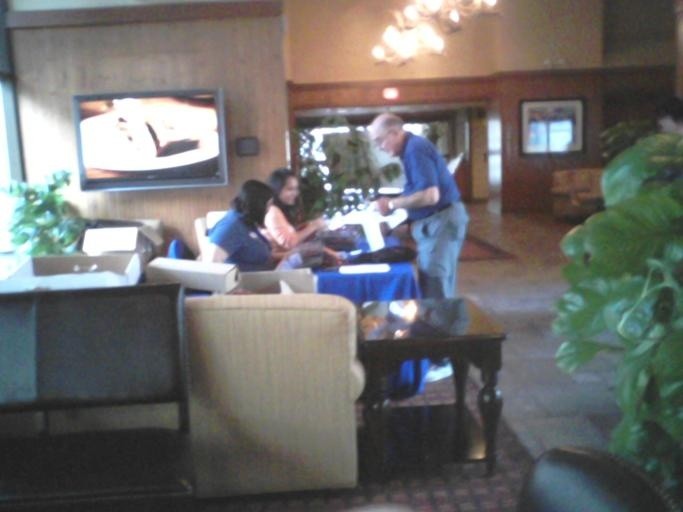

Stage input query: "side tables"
[{"left": 360, "top": 297, "right": 501, "bottom": 478}]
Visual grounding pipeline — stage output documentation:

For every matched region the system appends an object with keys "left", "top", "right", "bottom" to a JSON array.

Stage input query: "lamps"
[{"left": 370, "top": 0, "right": 498, "bottom": 61}]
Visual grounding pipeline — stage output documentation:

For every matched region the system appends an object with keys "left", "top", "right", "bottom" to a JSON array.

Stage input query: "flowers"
[{"left": 8, "top": 173, "right": 86, "bottom": 264}]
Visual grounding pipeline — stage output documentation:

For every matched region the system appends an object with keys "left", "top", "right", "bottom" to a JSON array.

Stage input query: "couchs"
[
  {"left": 194, "top": 209, "right": 247, "bottom": 271},
  {"left": 0, "top": 251, "right": 194, "bottom": 436},
  {"left": 179, "top": 288, "right": 363, "bottom": 492},
  {"left": 556, "top": 167, "right": 610, "bottom": 220}
]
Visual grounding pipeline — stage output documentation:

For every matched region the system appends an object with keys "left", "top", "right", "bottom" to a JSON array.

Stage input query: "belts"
[{"left": 410, "top": 200, "right": 456, "bottom": 220}]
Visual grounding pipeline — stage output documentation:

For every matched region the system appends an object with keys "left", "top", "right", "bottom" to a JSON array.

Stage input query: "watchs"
[{"left": 388, "top": 201, "right": 394, "bottom": 211}]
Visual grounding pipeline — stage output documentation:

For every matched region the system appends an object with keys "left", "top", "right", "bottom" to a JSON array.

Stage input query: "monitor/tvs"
[{"left": 71, "top": 88, "right": 228, "bottom": 192}]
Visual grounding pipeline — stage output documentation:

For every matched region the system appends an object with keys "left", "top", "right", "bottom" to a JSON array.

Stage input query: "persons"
[
  {"left": 207, "top": 179, "right": 343, "bottom": 273},
  {"left": 368, "top": 114, "right": 472, "bottom": 384},
  {"left": 263, "top": 169, "right": 328, "bottom": 264}
]
